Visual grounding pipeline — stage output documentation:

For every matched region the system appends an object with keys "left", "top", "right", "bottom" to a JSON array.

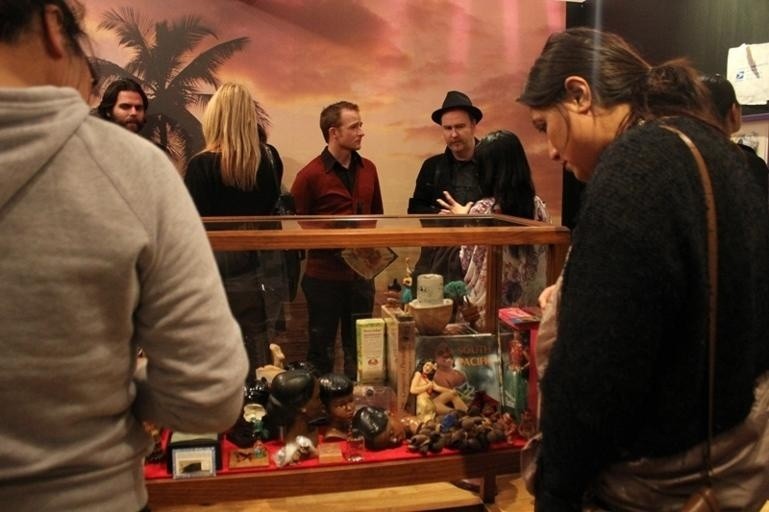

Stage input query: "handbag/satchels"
[{"left": 266, "top": 194, "right": 305, "bottom": 302}]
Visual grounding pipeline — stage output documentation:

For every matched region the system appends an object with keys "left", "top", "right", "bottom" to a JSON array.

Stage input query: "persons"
[
  {"left": 696, "top": 72, "right": 769, "bottom": 192},
  {"left": 185, "top": 80, "right": 284, "bottom": 372},
  {"left": 97, "top": 77, "right": 171, "bottom": 176},
  {"left": 515, "top": 25, "right": 769, "bottom": 512},
  {"left": 0, "top": 2, "right": 252, "bottom": 512},
  {"left": 269, "top": 331, "right": 535, "bottom": 468},
  {"left": 401, "top": 267, "right": 413, "bottom": 314},
  {"left": 289, "top": 101, "right": 384, "bottom": 382},
  {"left": 409, "top": 90, "right": 537, "bottom": 309},
  {"left": 384, "top": 277, "right": 402, "bottom": 310}
]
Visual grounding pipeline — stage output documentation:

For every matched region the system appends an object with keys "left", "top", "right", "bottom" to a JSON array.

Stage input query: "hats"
[{"left": 432, "top": 92, "right": 482, "bottom": 125}]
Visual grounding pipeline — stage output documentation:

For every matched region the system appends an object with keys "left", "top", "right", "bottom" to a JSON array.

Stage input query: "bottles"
[{"left": 345, "top": 429, "right": 366, "bottom": 463}]
